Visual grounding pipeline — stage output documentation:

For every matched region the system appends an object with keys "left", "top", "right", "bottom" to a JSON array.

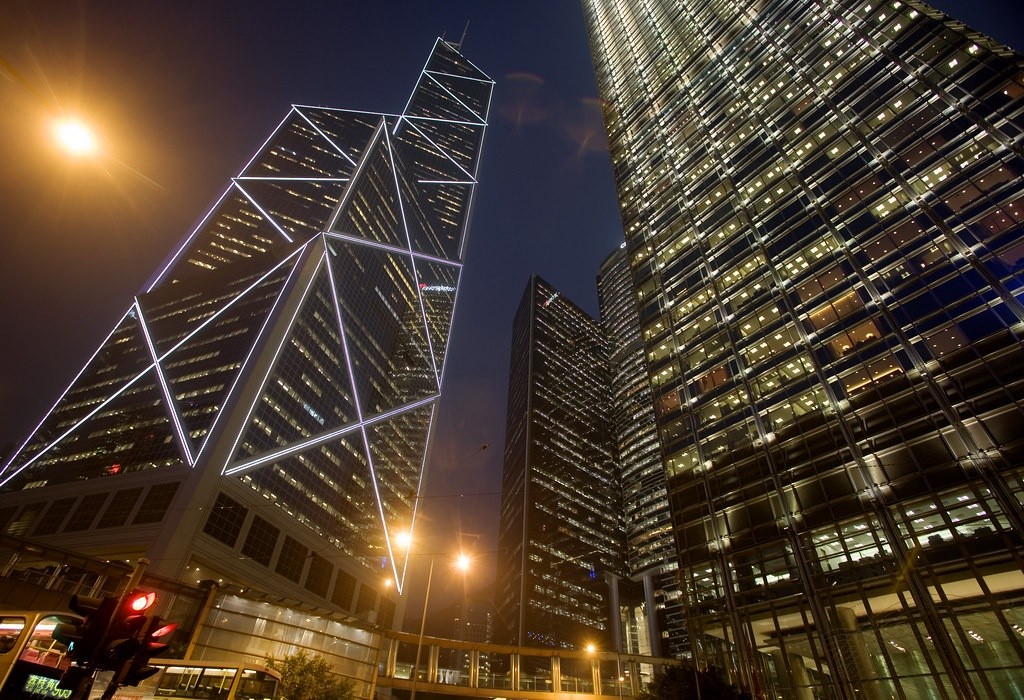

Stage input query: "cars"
[{"left": 607, "top": 671, "right": 642, "bottom": 696}]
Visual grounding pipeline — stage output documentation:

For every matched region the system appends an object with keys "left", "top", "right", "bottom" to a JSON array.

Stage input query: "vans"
[{"left": 394, "top": 662, "right": 428, "bottom": 682}]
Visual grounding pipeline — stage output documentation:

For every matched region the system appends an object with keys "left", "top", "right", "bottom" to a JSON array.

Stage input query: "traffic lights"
[
  {"left": 52, "top": 594, "right": 119, "bottom": 661},
  {"left": 95, "top": 589, "right": 157, "bottom": 671},
  {"left": 117, "top": 615, "right": 179, "bottom": 687}
]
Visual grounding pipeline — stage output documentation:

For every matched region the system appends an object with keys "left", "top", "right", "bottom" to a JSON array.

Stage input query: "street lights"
[{"left": 411, "top": 549, "right": 470, "bottom": 700}]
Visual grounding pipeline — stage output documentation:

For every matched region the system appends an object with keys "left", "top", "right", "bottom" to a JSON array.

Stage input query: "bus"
[
  {"left": 89, "top": 657, "right": 283, "bottom": 700},
  {"left": 0, "top": 611, "right": 99, "bottom": 700}
]
